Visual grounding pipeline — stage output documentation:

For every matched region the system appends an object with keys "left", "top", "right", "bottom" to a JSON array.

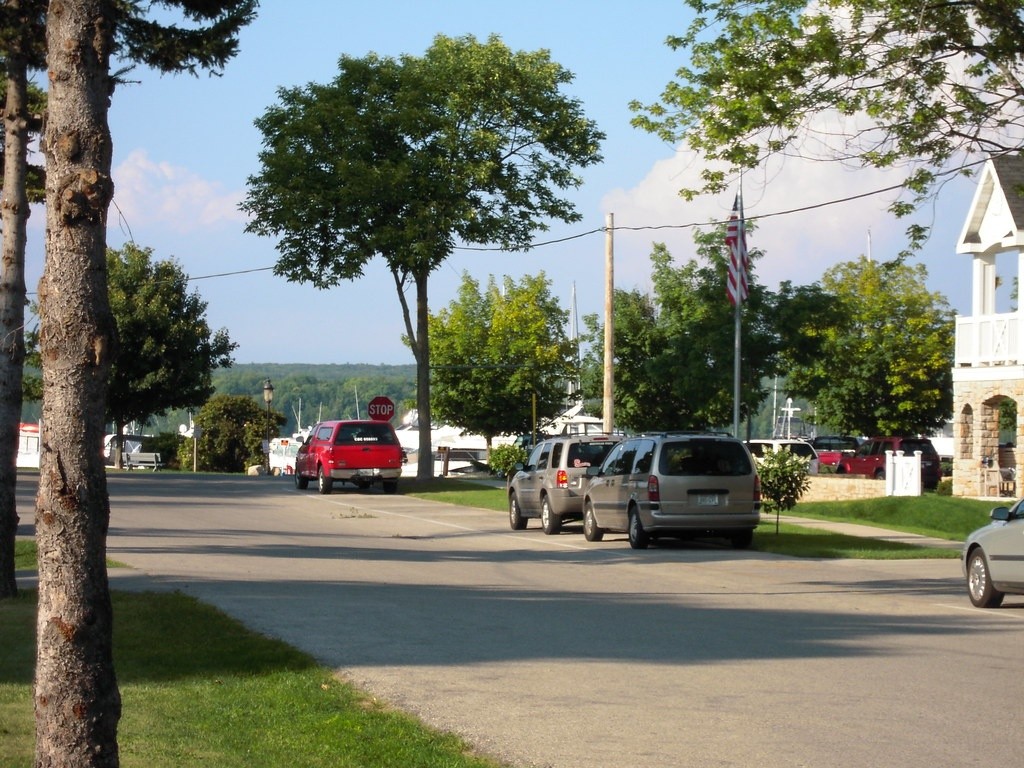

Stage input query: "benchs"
[{"left": 122, "top": 452, "right": 163, "bottom": 471}]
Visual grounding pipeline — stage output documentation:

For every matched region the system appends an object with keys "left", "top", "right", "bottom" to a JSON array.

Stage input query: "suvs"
[
  {"left": 506, "top": 429, "right": 624, "bottom": 537},
  {"left": 294, "top": 418, "right": 409, "bottom": 495},
  {"left": 833, "top": 434, "right": 943, "bottom": 491},
  {"left": 740, "top": 438, "right": 820, "bottom": 475},
  {"left": 581, "top": 430, "right": 764, "bottom": 550}
]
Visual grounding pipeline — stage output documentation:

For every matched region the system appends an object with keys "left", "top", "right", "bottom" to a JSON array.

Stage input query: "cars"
[
  {"left": 961, "top": 497, "right": 1023, "bottom": 610},
  {"left": 804, "top": 435, "right": 868, "bottom": 466}
]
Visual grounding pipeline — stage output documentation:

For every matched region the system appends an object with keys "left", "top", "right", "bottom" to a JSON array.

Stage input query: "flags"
[{"left": 725, "top": 191, "right": 750, "bottom": 305}]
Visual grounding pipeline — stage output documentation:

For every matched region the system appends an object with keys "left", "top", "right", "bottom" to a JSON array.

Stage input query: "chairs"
[{"left": 985, "top": 469, "right": 1016, "bottom": 498}]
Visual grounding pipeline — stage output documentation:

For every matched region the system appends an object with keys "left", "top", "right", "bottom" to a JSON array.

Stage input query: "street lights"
[{"left": 263, "top": 375, "right": 275, "bottom": 475}]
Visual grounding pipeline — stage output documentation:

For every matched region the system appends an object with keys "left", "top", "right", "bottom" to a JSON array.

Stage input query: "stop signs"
[{"left": 367, "top": 396, "right": 395, "bottom": 422}]
